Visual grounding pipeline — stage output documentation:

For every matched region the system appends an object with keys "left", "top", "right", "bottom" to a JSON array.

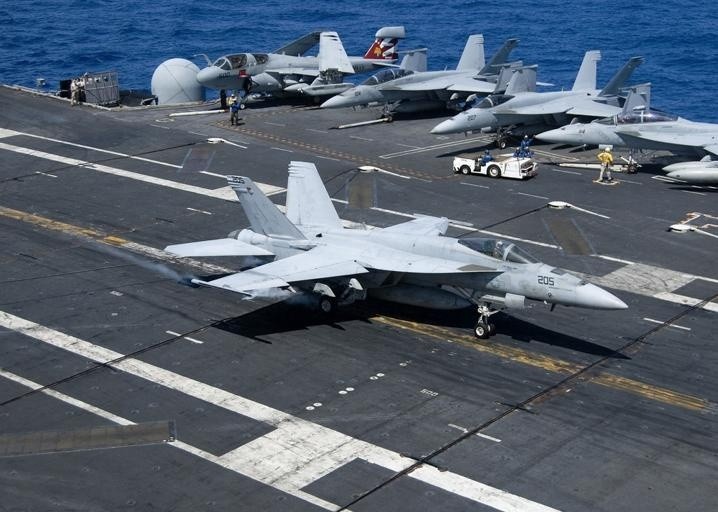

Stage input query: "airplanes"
[
  {"left": 661, "top": 154, "right": 718, "bottom": 193},
  {"left": 319, "top": 32, "right": 555, "bottom": 130},
  {"left": 532, "top": 82, "right": 717, "bottom": 160},
  {"left": 427, "top": 49, "right": 652, "bottom": 149},
  {"left": 197, "top": 25, "right": 429, "bottom": 110},
  {"left": 160, "top": 157, "right": 629, "bottom": 338}
]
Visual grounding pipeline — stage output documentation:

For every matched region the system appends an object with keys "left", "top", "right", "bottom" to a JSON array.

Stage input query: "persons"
[
  {"left": 519, "top": 134, "right": 534, "bottom": 149},
  {"left": 74, "top": 78, "right": 81, "bottom": 104},
  {"left": 595, "top": 148, "right": 613, "bottom": 182},
  {"left": 512, "top": 148, "right": 523, "bottom": 158},
  {"left": 229, "top": 93, "right": 237, "bottom": 122},
  {"left": 230, "top": 100, "right": 240, "bottom": 126},
  {"left": 520, "top": 147, "right": 534, "bottom": 158},
  {"left": 69, "top": 80, "right": 78, "bottom": 106},
  {"left": 478, "top": 149, "right": 496, "bottom": 166}
]
